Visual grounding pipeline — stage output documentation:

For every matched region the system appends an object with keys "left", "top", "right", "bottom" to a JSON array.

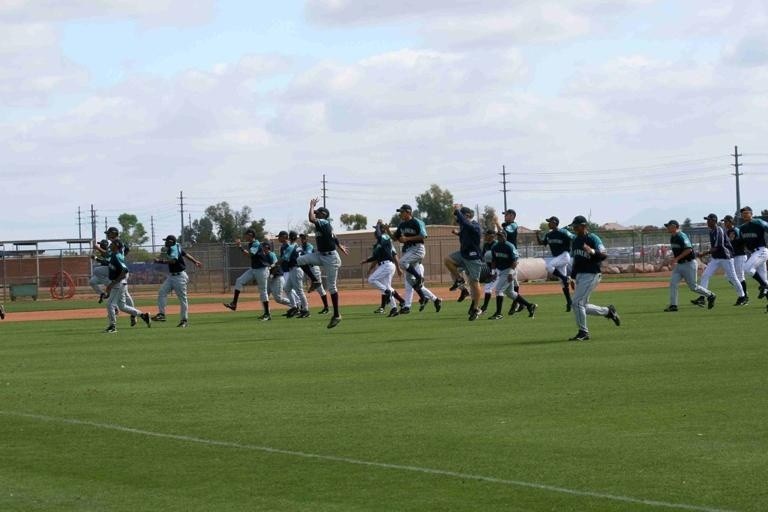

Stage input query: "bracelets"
[{"left": 94, "top": 256, "right": 97, "bottom": 260}]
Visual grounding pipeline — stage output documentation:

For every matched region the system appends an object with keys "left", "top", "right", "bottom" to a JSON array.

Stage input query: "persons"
[
  {"left": 443, "top": 204, "right": 483, "bottom": 321},
  {"left": 359, "top": 223, "right": 403, "bottom": 317},
  {"left": 397, "top": 262, "right": 443, "bottom": 314},
  {"left": 721, "top": 216, "right": 749, "bottom": 306},
  {"left": 296, "top": 196, "right": 350, "bottom": 328},
  {"left": 276, "top": 231, "right": 291, "bottom": 283},
  {"left": 691, "top": 214, "right": 746, "bottom": 307},
  {"left": 566, "top": 216, "right": 620, "bottom": 342},
  {"left": 88, "top": 239, "right": 109, "bottom": 303},
  {"left": 94, "top": 227, "right": 137, "bottom": 326},
  {"left": 664, "top": 220, "right": 716, "bottom": 311},
  {"left": 385, "top": 205, "right": 430, "bottom": 312},
  {"left": 103, "top": 240, "right": 151, "bottom": 333},
  {"left": 258, "top": 242, "right": 299, "bottom": 319},
  {"left": 152, "top": 235, "right": 202, "bottom": 327},
  {"left": 451, "top": 230, "right": 495, "bottom": 313},
  {"left": 223, "top": 229, "right": 273, "bottom": 321},
  {"left": 535, "top": 217, "right": 575, "bottom": 312},
  {"left": 367, "top": 231, "right": 386, "bottom": 313},
  {"left": 738, "top": 206, "right": 768, "bottom": 303},
  {"left": 275, "top": 231, "right": 311, "bottom": 318},
  {"left": 299, "top": 234, "right": 330, "bottom": 314},
  {"left": 501, "top": 210, "right": 520, "bottom": 315},
  {"left": 489, "top": 230, "right": 537, "bottom": 321}
]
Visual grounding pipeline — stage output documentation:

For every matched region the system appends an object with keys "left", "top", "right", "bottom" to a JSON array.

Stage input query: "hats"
[
  {"left": 721, "top": 215, "right": 733, "bottom": 222},
  {"left": 455, "top": 207, "right": 474, "bottom": 219},
  {"left": 314, "top": 208, "right": 329, "bottom": 217},
  {"left": 496, "top": 230, "right": 507, "bottom": 237},
  {"left": 664, "top": 220, "right": 679, "bottom": 228},
  {"left": 163, "top": 235, "right": 176, "bottom": 242},
  {"left": 704, "top": 214, "right": 717, "bottom": 220},
  {"left": 546, "top": 217, "right": 559, "bottom": 225},
  {"left": 275, "top": 231, "right": 288, "bottom": 237},
  {"left": 261, "top": 242, "right": 270, "bottom": 247},
  {"left": 483, "top": 230, "right": 497, "bottom": 236},
  {"left": 396, "top": 205, "right": 412, "bottom": 213},
  {"left": 97, "top": 240, "right": 108, "bottom": 246},
  {"left": 110, "top": 239, "right": 123, "bottom": 248},
  {"left": 502, "top": 210, "right": 516, "bottom": 215},
  {"left": 243, "top": 229, "right": 256, "bottom": 236},
  {"left": 104, "top": 227, "right": 119, "bottom": 235},
  {"left": 299, "top": 234, "right": 309, "bottom": 238},
  {"left": 568, "top": 216, "right": 587, "bottom": 226},
  {"left": 740, "top": 206, "right": 752, "bottom": 212}
]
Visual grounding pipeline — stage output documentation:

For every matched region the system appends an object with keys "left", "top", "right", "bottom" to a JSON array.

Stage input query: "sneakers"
[
  {"left": 399, "top": 300, "right": 406, "bottom": 310},
  {"left": 566, "top": 300, "right": 572, "bottom": 311},
  {"left": 691, "top": 296, "right": 705, "bottom": 307},
  {"left": 307, "top": 280, "right": 321, "bottom": 293},
  {"left": 664, "top": 305, "right": 678, "bottom": 311},
  {"left": 98, "top": 292, "right": 109, "bottom": 304},
  {"left": 296, "top": 310, "right": 311, "bottom": 318},
  {"left": 142, "top": 312, "right": 151, "bottom": 328},
  {"left": 374, "top": 308, "right": 385, "bottom": 313},
  {"left": 104, "top": 326, "right": 116, "bottom": 333},
  {"left": 224, "top": 303, "right": 237, "bottom": 311},
  {"left": 434, "top": 298, "right": 442, "bottom": 312},
  {"left": 151, "top": 313, "right": 166, "bottom": 321},
  {"left": 385, "top": 290, "right": 392, "bottom": 304},
  {"left": 733, "top": 297, "right": 748, "bottom": 306},
  {"left": 318, "top": 308, "right": 329, "bottom": 315},
  {"left": 469, "top": 308, "right": 482, "bottom": 321},
  {"left": 130, "top": 315, "right": 137, "bottom": 326},
  {"left": 481, "top": 306, "right": 487, "bottom": 312},
  {"left": 488, "top": 313, "right": 504, "bottom": 321},
  {"left": 257, "top": 313, "right": 271, "bottom": 321},
  {"left": 605, "top": 304, "right": 620, "bottom": 326},
  {"left": 457, "top": 288, "right": 470, "bottom": 302},
  {"left": 177, "top": 319, "right": 188, "bottom": 328},
  {"left": 528, "top": 304, "right": 538, "bottom": 318},
  {"left": 469, "top": 303, "right": 474, "bottom": 314},
  {"left": 758, "top": 286, "right": 768, "bottom": 299},
  {"left": 327, "top": 314, "right": 341, "bottom": 328},
  {"left": 386, "top": 308, "right": 398, "bottom": 317},
  {"left": 707, "top": 293, "right": 717, "bottom": 309},
  {"left": 399, "top": 307, "right": 410, "bottom": 314},
  {"left": 570, "top": 280, "right": 577, "bottom": 290},
  {"left": 449, "top": 277, "right": 465, "bottom": 291},
  {"left": 569, "top": 330, "right": 590, "bottom": 341},
  {"left": 287, "top": 309, "right": 298, "bottom": 318},
  {"left": 508, "top": 302, "right": 520, "bottom": 315},
  {"left": 115, "top": 308, "right": 119, "bottom": 315},
  {"left": 419, "top": 296, "right": 430, "bottom": 312}
]
